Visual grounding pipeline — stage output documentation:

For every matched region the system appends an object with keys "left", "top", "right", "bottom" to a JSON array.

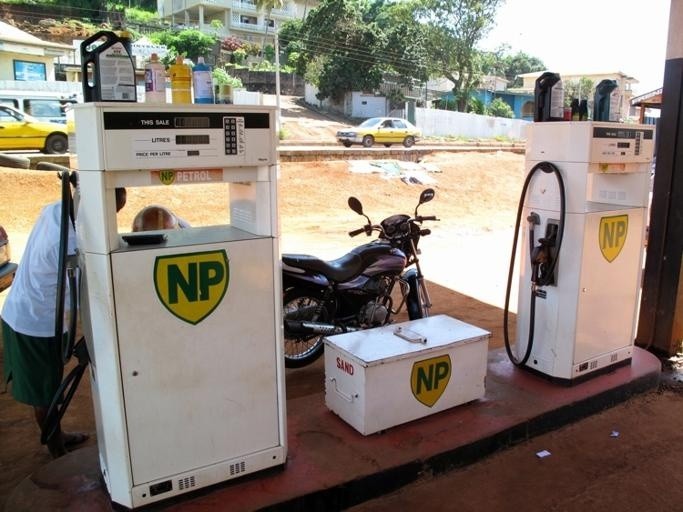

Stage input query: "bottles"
[
  {"left": 145, "top": 54, "right": 233, "bottom": 104},
  {"left": 563, "top": 98, "right": 588, "bottom": 121}
]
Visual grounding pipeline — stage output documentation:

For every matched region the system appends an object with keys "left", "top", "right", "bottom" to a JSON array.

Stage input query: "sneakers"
[{"left": 63, "top": 431, "right": 90, "bottom": 448}]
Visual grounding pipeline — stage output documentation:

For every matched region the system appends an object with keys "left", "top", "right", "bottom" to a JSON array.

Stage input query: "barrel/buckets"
[
  {"left": 79, "top": 26, "right": 138, "bottom": 103},
  {"left": 595, "top": 79, "right": 620, "bottom": 123},
  {"left": 534, "top": 71, "right": 563, "bottom": 122}
]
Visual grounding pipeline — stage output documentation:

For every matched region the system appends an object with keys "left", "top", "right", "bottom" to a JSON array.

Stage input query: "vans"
[{"left": 0, "top": 91, "right": 79, "bottom": 129}]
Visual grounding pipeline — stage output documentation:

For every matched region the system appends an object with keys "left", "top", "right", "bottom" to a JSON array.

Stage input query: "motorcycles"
[{"left": 280, "top": 189, "right": 439, "bottom": 368}]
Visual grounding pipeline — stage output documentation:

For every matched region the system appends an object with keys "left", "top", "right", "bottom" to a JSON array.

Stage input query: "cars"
[
  {"left": 336, "top": 116, "right": 422, "bottom": 148},
  {"left": 0, "top": 105, "right": 68, "bottom": 152}
]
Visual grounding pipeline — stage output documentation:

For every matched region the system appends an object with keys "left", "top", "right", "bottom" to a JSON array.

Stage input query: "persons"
[
  {"left": 1, "top": 183, "right": 128, "bottom": 461},
  {"left": 132, "top": 201, "right": 191, "bottom": 232}
]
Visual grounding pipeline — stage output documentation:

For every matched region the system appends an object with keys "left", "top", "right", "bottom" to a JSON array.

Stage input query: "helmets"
[{"left": 132, "top": 204, "right": 177, "bottom": 232}]
